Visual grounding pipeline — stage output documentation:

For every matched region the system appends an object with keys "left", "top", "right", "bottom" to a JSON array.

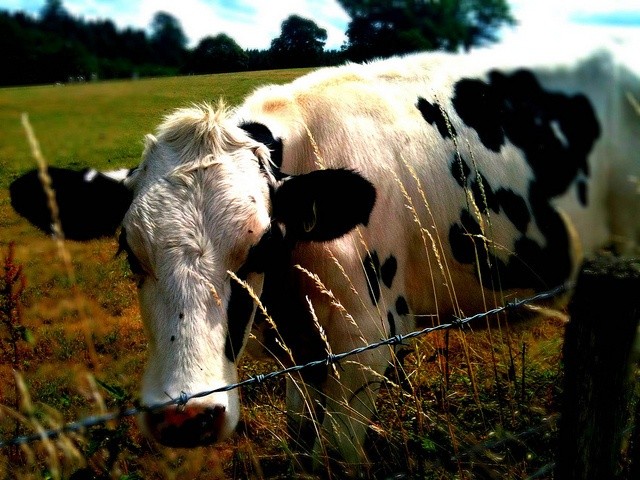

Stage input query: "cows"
[{"left": 9, "top": 35, "right": 628, "bottom": 472}]
